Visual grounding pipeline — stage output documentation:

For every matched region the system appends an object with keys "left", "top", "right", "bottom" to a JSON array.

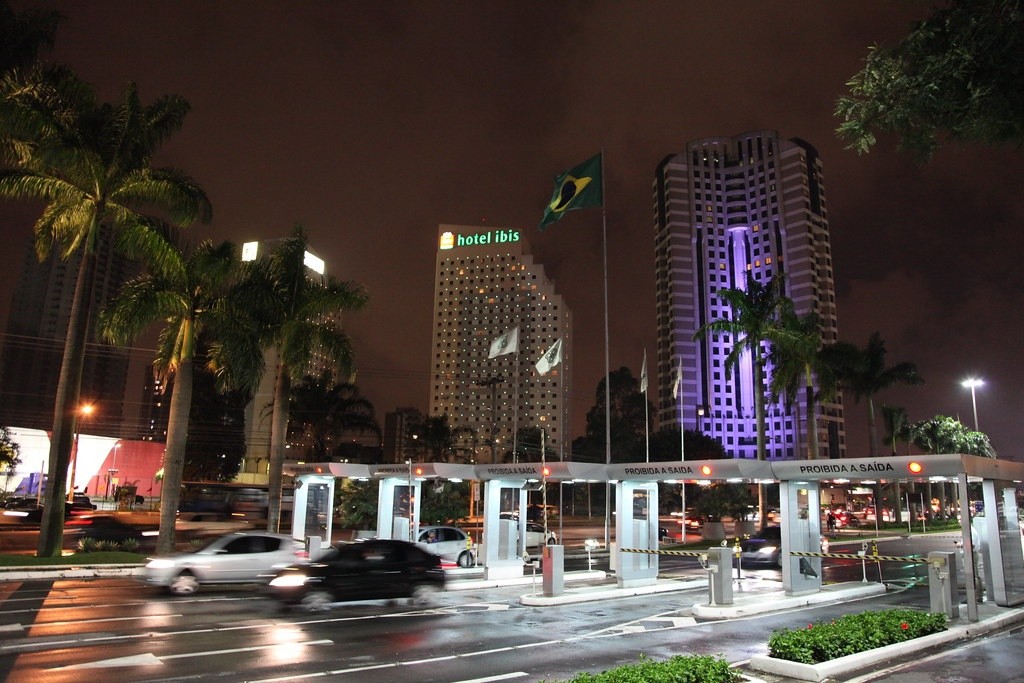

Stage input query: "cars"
[
  {"left": 145, "top": 529, "right": 299, "bottom": 596},
  {"left": 736, "top": 525, "right": 829, "bottom": 567},
  {"left": 268, "top": 538, "right": 447, "bottom": 613},
  {"left": 835, "top": 514, "right": 861, "bottom": 528},
  {"left": 500, "top": 512, "right": 522, "bottom": 524},
  {"left": 0, "top": 492, "right": 43, "bottom": 524},
  {"left": 414, "top": 526, "right": 474, "bottom": 569},
  {"left": 65, "top": 493, "right": 98, "bottom": 510},
  {"left": 681, "top": 514, "right": 705, "bottom": 535}
]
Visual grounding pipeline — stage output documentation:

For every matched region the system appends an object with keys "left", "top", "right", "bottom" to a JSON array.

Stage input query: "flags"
[
  {"left": 671, "top": 357, "right": 682, "bottom": 399},
  {"left": 534, "top": 335, "right": 563, "bottom": 375},
  {"left": 537, "top": 152, "right": 604, "bottom": 235},
  {"left": 488, "top": 325, "right": 521, "bottom": 357},
  {"left": 639, "top": 348, "right": 649, "bottom": 392}
]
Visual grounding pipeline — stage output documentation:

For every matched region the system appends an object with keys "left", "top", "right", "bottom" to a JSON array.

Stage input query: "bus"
[{"left": 177, "top": 481, "right": 294, "bottom": 523}]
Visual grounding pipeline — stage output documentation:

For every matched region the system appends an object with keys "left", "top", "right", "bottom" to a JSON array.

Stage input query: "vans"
[
  {"left": 866, "top": 505, "right": 910, "bottom": 523},
  {"left": 517, "top": 521, "right": 557, "bottom": 548}
]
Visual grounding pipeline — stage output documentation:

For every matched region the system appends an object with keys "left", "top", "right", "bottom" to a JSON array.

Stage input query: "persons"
[
  {"left": 422, "top": 529, "right": 438, "bottom": 544},
  {"left": 825, "top": 505, "right": 836, "bottom": 540}
]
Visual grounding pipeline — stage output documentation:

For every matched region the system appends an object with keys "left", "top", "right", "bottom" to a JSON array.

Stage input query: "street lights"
[
  {"left": 110, "top": 443, "right": 122, "bottom": 498},
  {"left": 963, "top": 377, "right": 982, "bottom": 432},
  {"left": 67, "top": 404, "right": 93, "bottom": 502}
]
